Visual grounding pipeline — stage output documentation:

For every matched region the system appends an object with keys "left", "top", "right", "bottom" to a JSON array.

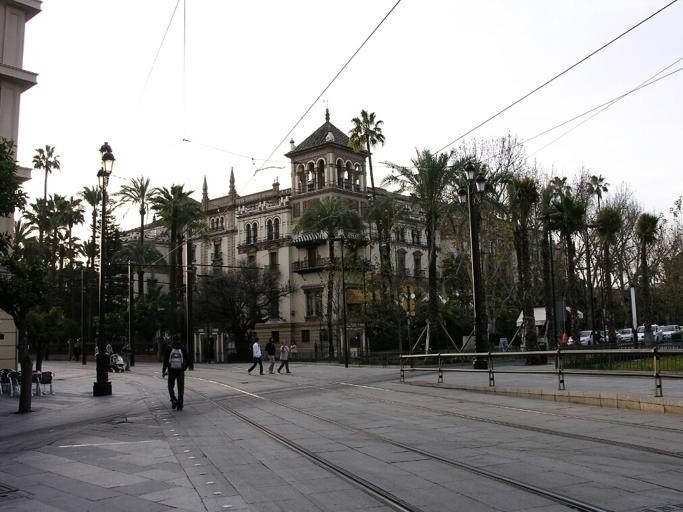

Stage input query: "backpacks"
[{"left": 168, "top": 344, "right": 184, "bottom": 368}]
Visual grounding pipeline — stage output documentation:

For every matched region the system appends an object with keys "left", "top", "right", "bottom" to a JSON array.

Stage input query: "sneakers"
[
  {"left": 248, "top": 370, "right": 291, "bottom": 375},
  {"left": 172, "top": 400, "right": 177, "bottom": 410}
]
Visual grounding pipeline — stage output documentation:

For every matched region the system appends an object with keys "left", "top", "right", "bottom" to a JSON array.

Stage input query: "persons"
[
  {"left": 105, "top": 343, "right": 113, "bottom": 356},
  {"left": 265, "top": 338, "right": 276, "bottom": 374},
  {"left": 122, "top": 343, "right": 132, "bottom": 371},
  {"left": 278, "top": 341, "right": 291, "bottom": 373},
  {"left": 73, "top": 338, "right": 82, "bottom": 361},
  {"left": 248, "top": 337, "right": 265, "bottom": 376},
  {"left": 162, "top": 334, "right": 190, "bottom": 411},
  {"left": 290, "top": 340, "right": 298, "bottom": 362}
]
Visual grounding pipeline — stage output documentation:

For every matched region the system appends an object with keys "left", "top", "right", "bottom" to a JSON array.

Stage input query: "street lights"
[
  {"left": 92, "top": 143, "right": 114, "bottom": 397},
  {"left": 337, "top": 228, "right": 348, "bottom": 368},
  {"left": 458, "top": 159, "right": 490, "bottom": 370}
]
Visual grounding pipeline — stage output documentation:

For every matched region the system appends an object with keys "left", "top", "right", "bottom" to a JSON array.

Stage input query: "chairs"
[{"left": 0, "top": 368, "right": 56, "bottom": 397}]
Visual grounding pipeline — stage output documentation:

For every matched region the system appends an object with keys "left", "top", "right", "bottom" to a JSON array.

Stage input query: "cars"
[{"left": 566, "top": 323, "right": 683, "bottom": 347}]
